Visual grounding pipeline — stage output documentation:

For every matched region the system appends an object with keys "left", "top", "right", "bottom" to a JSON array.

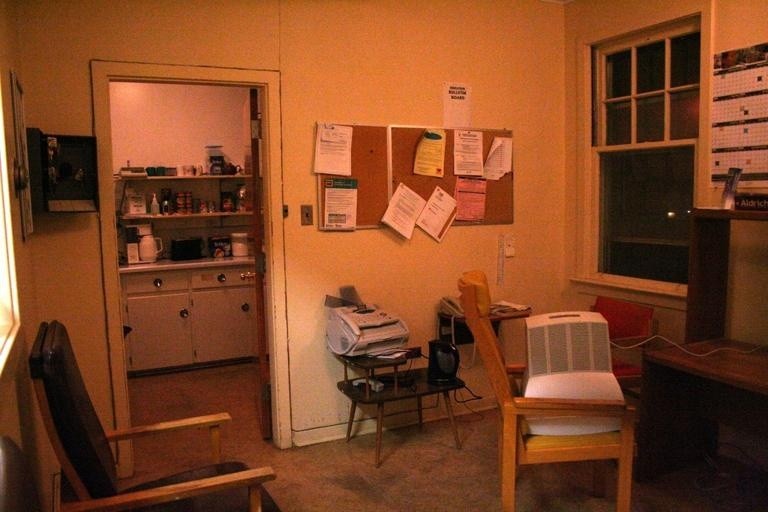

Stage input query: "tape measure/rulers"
[{"left": 497, "top": 233, "right": 504, "bottom": 285}]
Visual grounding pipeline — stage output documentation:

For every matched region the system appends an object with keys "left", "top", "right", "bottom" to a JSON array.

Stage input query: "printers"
[{"left": 326, "top": 301, "right": 410, "bottom": 358}]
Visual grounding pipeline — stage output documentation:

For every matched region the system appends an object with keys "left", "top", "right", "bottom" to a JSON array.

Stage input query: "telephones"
[{"left": 440, "top": 296, "right": 465, "bottom": 319}]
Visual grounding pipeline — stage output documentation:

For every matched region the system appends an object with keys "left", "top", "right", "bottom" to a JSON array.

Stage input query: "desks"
[
  {"left": 643, "top": 208, "right": 768, "bottom": 478},
  {"left": 333, "top": 354, "right": 467, "bottom": 469}
]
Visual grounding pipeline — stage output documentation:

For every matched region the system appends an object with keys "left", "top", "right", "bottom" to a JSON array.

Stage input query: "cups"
[{"left": 146, "top": 166, "right": 166, "bottom": 176}]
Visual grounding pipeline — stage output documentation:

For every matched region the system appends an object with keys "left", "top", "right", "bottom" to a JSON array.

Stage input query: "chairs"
[{"left": 25, "top": 318, "right": 281, "bottom": 512}]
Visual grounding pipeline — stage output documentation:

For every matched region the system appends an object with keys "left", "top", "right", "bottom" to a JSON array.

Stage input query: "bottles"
[{"left": 230, "top": 231, "right": 250, "bottom": 256}]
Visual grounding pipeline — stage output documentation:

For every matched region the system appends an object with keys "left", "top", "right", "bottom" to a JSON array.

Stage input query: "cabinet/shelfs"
[
  {"left": 115, "top": 172, "right": 254, "bottom": 221},
  {"left": 119, "top": 264, "right": 259, "bottom": 378},
  {"left": 458, "top": 269, "right": 640, "bottom": 512}
]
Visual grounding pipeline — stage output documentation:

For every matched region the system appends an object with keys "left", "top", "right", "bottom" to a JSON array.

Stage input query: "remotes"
[{"left": 352, "top": 377, "right": 384, "bottom": 393}]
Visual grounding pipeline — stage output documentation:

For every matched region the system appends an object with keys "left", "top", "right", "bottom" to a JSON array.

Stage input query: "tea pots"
[{"left": 138, "top": 236, "right": 163, "bottom": 262}]
